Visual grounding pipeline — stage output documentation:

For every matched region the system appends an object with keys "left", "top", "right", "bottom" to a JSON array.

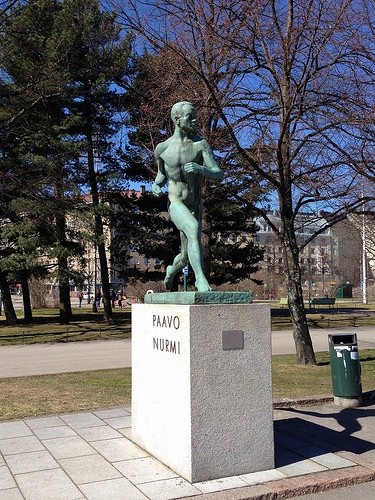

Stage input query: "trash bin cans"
[{"left": 327, "top": 332, "right": 365, "bottom": 406}]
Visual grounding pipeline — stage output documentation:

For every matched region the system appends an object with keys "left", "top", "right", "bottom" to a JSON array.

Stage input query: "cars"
[{"left": 82, "top": 291, "right": 95, "bottom": 300}]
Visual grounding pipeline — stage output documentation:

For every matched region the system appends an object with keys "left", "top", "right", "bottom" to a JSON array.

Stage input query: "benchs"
[
  {"left": 310, "top": 298, "right": 336, "bottom": 313},
  {"left": 278, "top": 298, "right": 289, "bottom": 308}
]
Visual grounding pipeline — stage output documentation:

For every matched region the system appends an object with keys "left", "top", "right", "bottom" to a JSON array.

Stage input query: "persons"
[
  {"left": 264, "top": 290, "right": 269, "bottom": 299},
  {"left": 78, "top": 288, "right": 124, "bottom": 308},
  {"left": 269, "top": 290, "right": 278, "bottom": 299},
  {"left": 152, "top": 101, "right": 222, "bottom": 292}
]
